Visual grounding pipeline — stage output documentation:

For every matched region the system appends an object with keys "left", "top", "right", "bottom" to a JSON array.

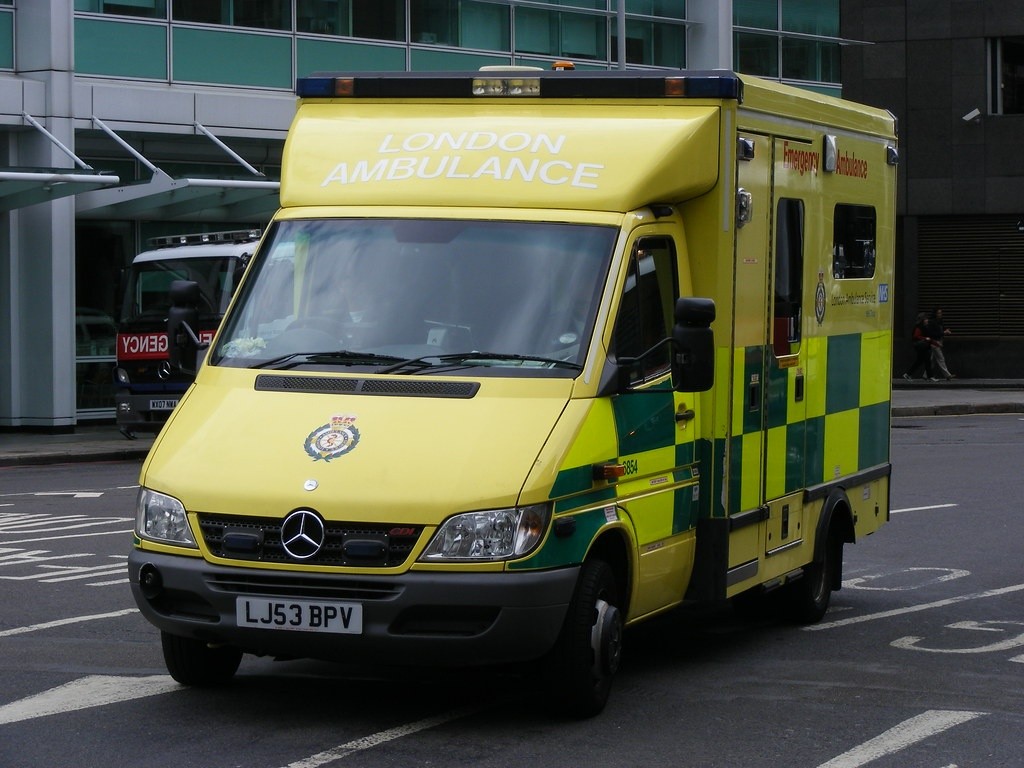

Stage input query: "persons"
[
  {"left": 922, "top": 307, "right": 956, "bottom": 380},
  {"left": 334, "top": 269, "right": 379, "bottom": 327},
  {"left": 903, "top": 318, "right": 943, "bottom": 381}
]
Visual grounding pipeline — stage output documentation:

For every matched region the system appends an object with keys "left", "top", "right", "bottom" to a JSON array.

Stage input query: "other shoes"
[
  {"left": 904, "top": 373, "right": 912, "bottom": 383},
  {"left": 946, "top": 374, "right": 955, "bottom": 381},
  {"left": 927, "top": 377, "right": 938, "bottom": 383}
]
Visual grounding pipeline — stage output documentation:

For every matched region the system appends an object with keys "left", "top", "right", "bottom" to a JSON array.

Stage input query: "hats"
[{"left": 917, "top": 313, "right": 927, "bottom": 324}]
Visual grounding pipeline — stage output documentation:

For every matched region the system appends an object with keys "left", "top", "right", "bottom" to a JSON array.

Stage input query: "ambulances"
[
  {"left": 109, "top": 230, "right": 297, "bottom": 438},
  {"left": 126, "top": 63, "right": 895, "bottom": 723}
]
